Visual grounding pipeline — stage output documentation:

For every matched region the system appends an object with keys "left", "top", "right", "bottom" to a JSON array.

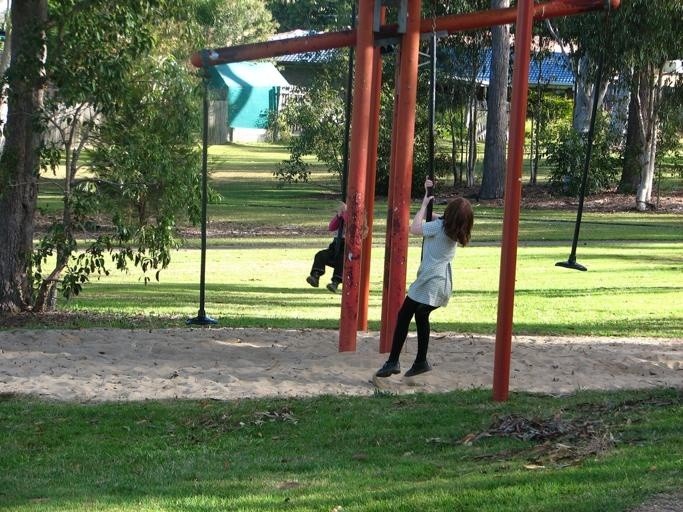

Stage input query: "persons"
[
  {"left": 375, "top": 175, "right": 476, "bottom": 378},
  {"left": 305, "top": 200, "right": 368, "bottom": 293}
]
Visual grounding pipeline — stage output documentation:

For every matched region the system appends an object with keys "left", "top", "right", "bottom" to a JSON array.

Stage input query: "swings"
[
  {"left": 556, "top": 1, "right": 610, "bottom": 271},
  {"left": 329, "top": 0, "right": 356, "bottom": 269},
  {"left": 417, "top": 0, "right": 436, "bottom": 314}
]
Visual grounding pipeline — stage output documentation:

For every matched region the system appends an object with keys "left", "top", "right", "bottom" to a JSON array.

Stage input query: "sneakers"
[
  {"left": 375, "top": 361, "right": 401, "bottom": 378},
  {"left": 307, "top": 276, "right": 319, "bottom": 287},
  {"left": 327, "top": 284, "right": 337, "bottom": 293},
  {"left": 404, "top": 360, "right": 431, "bottom": 377}
]
